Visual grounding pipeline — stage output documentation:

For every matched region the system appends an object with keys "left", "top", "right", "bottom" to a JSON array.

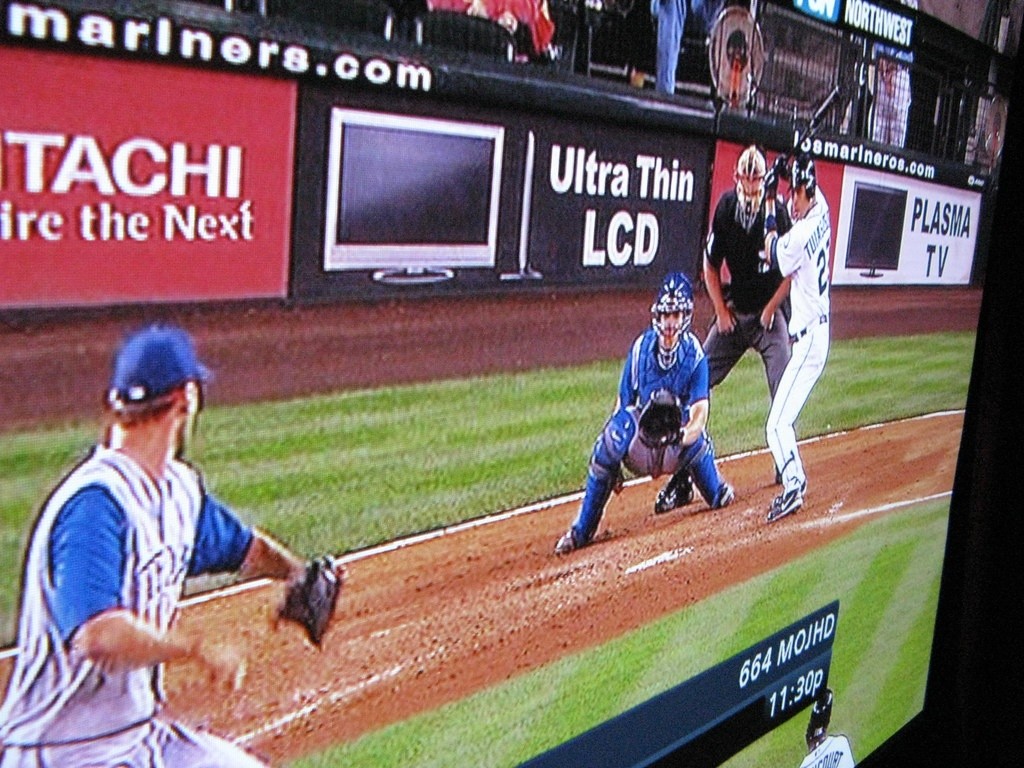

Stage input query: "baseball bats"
[{"left": 763, "top": 85, "right": 844, "bottom": 188}]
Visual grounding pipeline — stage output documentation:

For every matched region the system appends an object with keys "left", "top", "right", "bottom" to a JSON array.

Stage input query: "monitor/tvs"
[
  {"left": 323, "top": 107, "right": 506, "bottom": 284},
  {"left": 845, "top": 182, "right": 908, "bottom": 278}
]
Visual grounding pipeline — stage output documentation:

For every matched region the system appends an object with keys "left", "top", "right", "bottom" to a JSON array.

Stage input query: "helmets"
[
  {"left": 737, "top": 144, "right": 766, "bottom": 213},
  {"left": 811, "top": 688, "right": 833, "bottom": 726},
  {"left": 655, "top": 270, "right": 693, "bottom": 312},
  {"left": 790, "top": 152, "right": 816, "bottom": 199}
]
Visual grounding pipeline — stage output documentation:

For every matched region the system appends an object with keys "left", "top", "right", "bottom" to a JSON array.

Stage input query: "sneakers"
[
  {"left": 714, "top": 481, "right": 733, "bottom": 508},
  {"left": 655, "top": 474, "right": 693, "bottom": 512},
  {"left": 555, "top": 530, "right": 595, "bottom": 551},
  {"left": 764, "top": 489, "right": 804, "bottom": 523}
]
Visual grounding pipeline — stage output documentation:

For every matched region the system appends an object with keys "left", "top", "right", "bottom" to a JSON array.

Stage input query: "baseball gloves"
[
  {"left": 270, "top": 554, "right": 347, "bottom": 653},
  {"left": 636, "top": 394, "right": 683, "bottom": 448}
]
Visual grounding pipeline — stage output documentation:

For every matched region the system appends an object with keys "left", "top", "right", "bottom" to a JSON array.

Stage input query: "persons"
[
  {"left": 760, "top": 151, "right": 832, "bottom": 523},
  {"left": 800, "top": 689, "right": 855, "bottom": 768},
  {"left": 652, "top": 145, "right": 795, "bottom": 514},
  {"left": 0, "top": 330, "right": 348, "bottom": 768},
  {"left": 555, "top": 273, "right": 735, "bottom": 554},
  {"left": 651, "top": 0, "right": 687, "bottom": 94}
]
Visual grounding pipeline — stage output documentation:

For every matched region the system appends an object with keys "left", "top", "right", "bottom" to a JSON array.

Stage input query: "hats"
[{"left": 108, "top": 324, "right": 214, "bottom": 398}]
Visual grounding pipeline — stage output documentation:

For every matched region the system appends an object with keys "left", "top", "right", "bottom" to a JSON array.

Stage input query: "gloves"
[{"left": 764, "top": 154, "right": 789, "bottom": 197}]
